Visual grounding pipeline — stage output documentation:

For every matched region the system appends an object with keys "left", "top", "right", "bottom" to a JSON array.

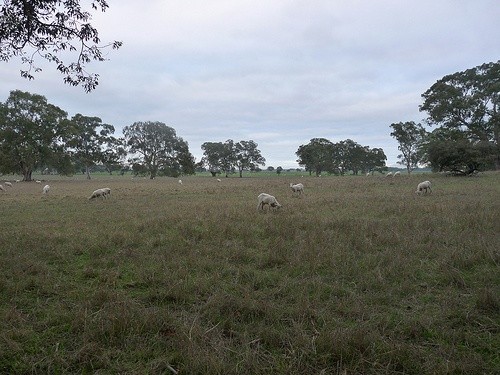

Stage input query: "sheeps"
[
  {"left": 88, "top": 188, "right": 111, "bottom": 201},
  {"left": 217, "top": 178, "right": 221, "bottom": 182},
  {"left": 44, "top": 184, "right": 50, "bottom": 194},
  {"left": 366, "top": 170, "right": 452, "bottom": 179},
  {"left": 0, "top": 180, "right": 41, "bottom": 192},
  {"left": 416, "top": 181, "right": 432, "bottom": 194},
  {"left": 290, "top": 182, "right": 305, "bottom": 197},
  {"left": 179, "top": 179, "right": 182, "bottom": 184},
  {"left": 257, "top": 193, "right": 281, "bottom": 213}
]
